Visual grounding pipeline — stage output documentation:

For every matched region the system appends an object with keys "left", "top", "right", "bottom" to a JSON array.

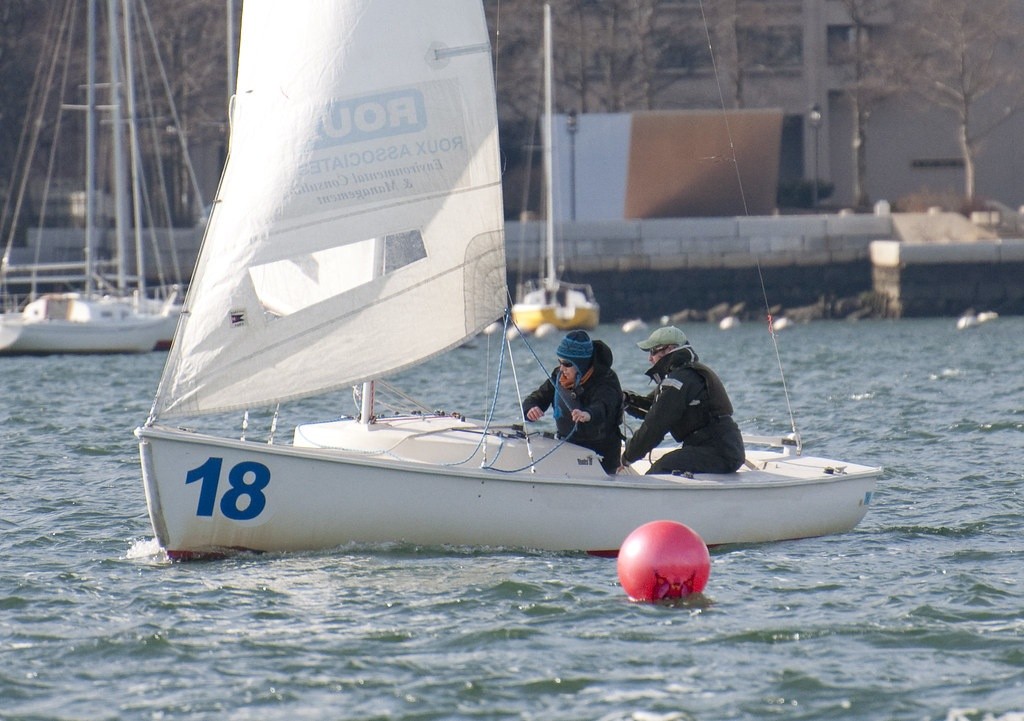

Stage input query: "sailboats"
[
  {"left": 134, "top": 0, "right": 885, "bottom": 564},
  {"left": 1, "top": 1, "right": 207, "bottom": 357},
  {"left": 510, "top": 1, "right": 602, "bottom": 330}
]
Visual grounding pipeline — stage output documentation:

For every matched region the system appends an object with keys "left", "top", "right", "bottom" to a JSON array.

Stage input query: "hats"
[
  {"left": 638, "top": 326, "right": 688, "bottom": 351},
  {"left": 557, "top": 331, "right": 594, "bottom": 377}
]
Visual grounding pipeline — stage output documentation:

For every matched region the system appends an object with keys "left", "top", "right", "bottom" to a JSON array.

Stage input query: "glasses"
[
  {"left": 558, "top": 359, "right": 572, "bottom": 367},
  {"left": 649, "top": 345, "right": 669, "bottom": 355}
]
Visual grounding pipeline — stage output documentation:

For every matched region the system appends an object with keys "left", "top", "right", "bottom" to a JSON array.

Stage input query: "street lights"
[
  {"left": 565, "top": 108, "right": 578, "bottom": 220},
  {"left": 809, "top": 104, "right": 822, "bottom": 209}
]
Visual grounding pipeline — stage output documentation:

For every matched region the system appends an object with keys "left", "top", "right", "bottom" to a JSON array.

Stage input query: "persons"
[
  {"left": 522, "top": 331, "right": 622, "bottom": 474},
  {"left": 619, "top": 325, "right": 747, "bottom": 475}
]
